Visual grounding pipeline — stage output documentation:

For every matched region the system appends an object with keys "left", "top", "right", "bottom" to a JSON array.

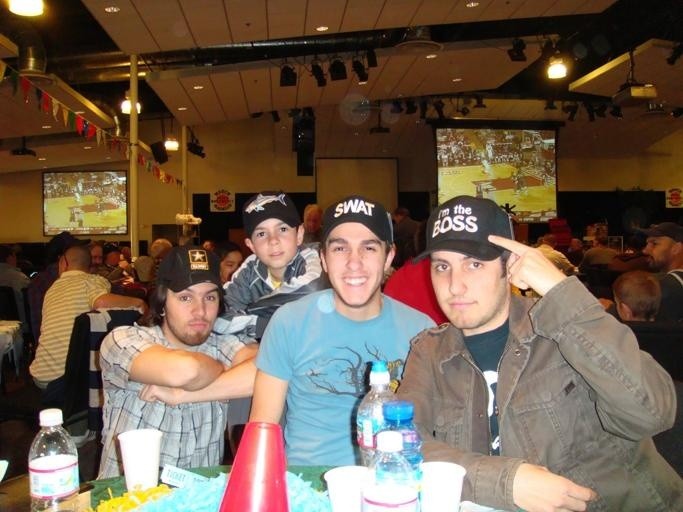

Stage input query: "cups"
[
  {"left": 420, "top": 461, "right": 467, "bottom": 512},
  {"left": 324, "top": 465, "right": 366, "bottom": 512},
  {"left": 118, "top": 429, "right": 163, "bottom": 493},
  {"left": 218, "top": 421, "right": 289, "bottom": 512}
]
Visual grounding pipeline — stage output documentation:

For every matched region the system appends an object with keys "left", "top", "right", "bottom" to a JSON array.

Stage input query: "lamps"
[
  {"left": 122, "top": 89, "right": 141, "bottom": 115},
  {"left": 162, "top": 115, "right": 178, "bottom": 151}
]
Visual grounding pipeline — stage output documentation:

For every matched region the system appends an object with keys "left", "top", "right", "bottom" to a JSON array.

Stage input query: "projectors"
[
  {"left": 370, "top": 127, "right": 390, "bottom": 133},
  {"left": 611, "top": 86, "right": 657, "bottom": 108},
  {"left": 9, "top": 148, "right": 36, "bottom": 159}
]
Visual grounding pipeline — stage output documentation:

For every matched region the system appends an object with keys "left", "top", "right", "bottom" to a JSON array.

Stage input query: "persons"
[
  {"left": 2, "top": 205, "right": 320, "bottom": 422},
  {"left": 44, "top": 172, "right": 127, "bottom": 234},
  {"left": 437, "top": 129, "right": 556, "bottom": 210},
  {"left": 96, "top": 245, "right": 258, "bottom": 480},
  {"left": 527, "top": 219, "right": 683, "bottom": 383},
  {"left": 249, "top": 196, "right": 436, "bottom": 468},
  {"left": 218, "top": 190, "right": 329, "bottom": 346},
  {"left": 395, "top": 194, "right": 683, "bottom": 512}
]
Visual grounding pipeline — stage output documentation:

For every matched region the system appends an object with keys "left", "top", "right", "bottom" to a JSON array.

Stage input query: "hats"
[
  {"left": 412, "top": 196, "right": 515, "bottom": 265},
  {"left": 47, "top": 231, "right": 91, "bottom": 261},
  {"left": 240, "top": 193, "right": 302, "bottom": 236},
  {"left": 320, "top": 196, "right": 392, "bottom": 242},
  {"left": 154, "top": 246, "right": 223, "bottom": 294},
  {"left": 646, "top": 223, "right": 683, "bottom": 243},
  {"left": 134, "top": 255, "right": 155, "bottom": 282}
]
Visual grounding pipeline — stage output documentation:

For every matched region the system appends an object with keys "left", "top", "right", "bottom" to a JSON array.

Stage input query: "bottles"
[
  {"left": 119, "top": 254, "right": 128, "bottom": 270},
  {"left": 131, "top": 258, "right": 135, "bottom": 268},
  {"left": 379, "top": 400, "right": 423, "bottom": 511},
  {"left": 362, "top": 432, "right": 419, "bottom": 512},
  {"left": 357, "top": 360, "right": 397, "bottom": 466},
  {"left": 28, "top": 409, "right": 80, "bottom": 512}
]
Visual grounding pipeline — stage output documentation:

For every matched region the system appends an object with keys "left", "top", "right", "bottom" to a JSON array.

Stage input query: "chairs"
[{"left": 0, "top": 246, "right": 148, "bottom": 511}]
